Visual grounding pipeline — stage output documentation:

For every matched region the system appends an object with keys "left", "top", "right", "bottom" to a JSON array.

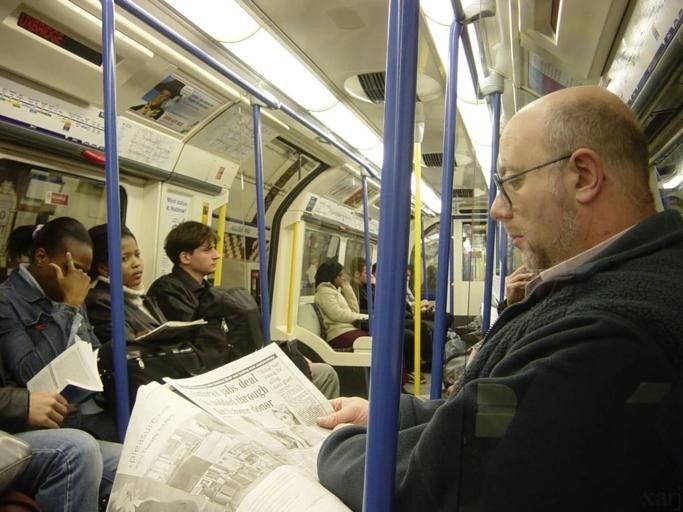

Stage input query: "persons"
[
  {"left": 313, "top": 258, "right": 413, "bottom": 394},
  {"left": 0, "top": 217, "right": 117, "bottom": 440},
  {"left": 106, "top": 485, "right": 198, "bottom": 512},
  {"left": 0, "top": 352, "right": 124, "bottom": 512},
  {"left": 348, "top": 259, "right": 485, "bottom": 395},
  {"left": 127, "top": 76, "right": 185, "bottom": 123},
  {"left": 80, "top": 222, "right": 171, "bottom": 431},
  {"left": 271, "top": 408, "right": 325, "bottom": 448},
  {"left": 145, "top": 219, "right": 341, "bottom": 398},
  {"left": 495, "top": 264, "right": 537, "bottom": 316},
  {"left": 314, "top": 85, "right": 682, "bottom": 511}
]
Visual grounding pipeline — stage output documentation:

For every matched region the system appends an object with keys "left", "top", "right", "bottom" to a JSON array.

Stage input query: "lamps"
[{"left": 159, "top": 0, "right": 499, "bottom": 217}]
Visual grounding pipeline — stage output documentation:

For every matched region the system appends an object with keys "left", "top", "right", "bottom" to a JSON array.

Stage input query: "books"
[
  {"left": 132, "top": 318, "right": 208, "bottom": 344},
  {"left": 24, "top": 342, "right": 104, "bottom": 409}
]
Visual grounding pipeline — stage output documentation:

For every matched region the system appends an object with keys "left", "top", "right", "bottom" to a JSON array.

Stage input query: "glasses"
[{"left": 493, "top": 155, "right": 571, "bottom": 207}]
